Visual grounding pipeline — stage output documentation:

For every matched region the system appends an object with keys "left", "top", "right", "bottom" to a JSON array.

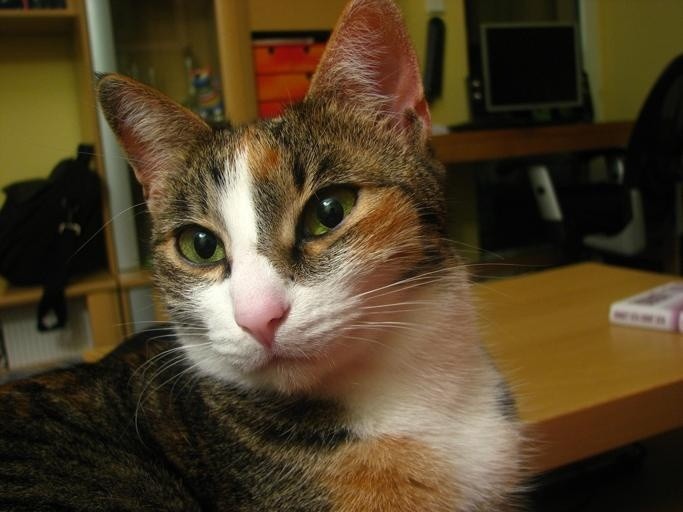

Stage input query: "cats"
[{"left": 1, "top": 0, "right": 542, "bottom": 511}]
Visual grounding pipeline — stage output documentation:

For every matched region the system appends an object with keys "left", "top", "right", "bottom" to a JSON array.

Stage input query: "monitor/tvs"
[{"left": 480, "top": 20, "right": 583, "bottom": 119}]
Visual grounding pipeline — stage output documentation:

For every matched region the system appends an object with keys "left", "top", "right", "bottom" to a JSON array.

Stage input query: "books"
[{"left": 607, "top": 275, "right": 683, "bottom": 336}]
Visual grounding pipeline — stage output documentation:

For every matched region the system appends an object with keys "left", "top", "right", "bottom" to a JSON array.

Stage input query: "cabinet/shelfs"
[{"left": 2, "top": 1, "right": 265, "bottom": 386}]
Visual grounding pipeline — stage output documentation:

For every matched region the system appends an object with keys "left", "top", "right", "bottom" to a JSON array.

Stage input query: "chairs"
[{"left": 549, "top": 58, "right": 680, "bottom": 271}]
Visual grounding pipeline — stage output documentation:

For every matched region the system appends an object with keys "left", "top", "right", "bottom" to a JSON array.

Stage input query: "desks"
[
  {"left": 460, "top": 262, "right": 681, "bottom": 512},
  {"left": 424, "top": 117, "right": 636, "bottom": 229}
]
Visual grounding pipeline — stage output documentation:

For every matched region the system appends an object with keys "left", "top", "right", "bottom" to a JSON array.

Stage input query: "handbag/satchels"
[{"left": 1, "top": 141, "right": 106, "bottom": 330}]
